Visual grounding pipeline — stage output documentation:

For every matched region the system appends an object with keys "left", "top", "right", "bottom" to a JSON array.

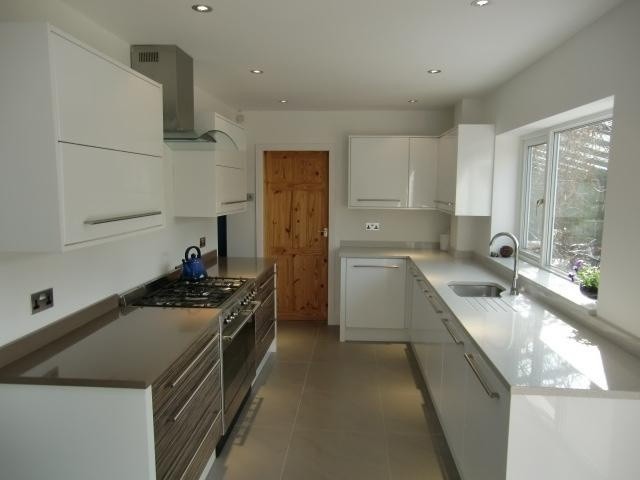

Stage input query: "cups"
[{"left": 439, "top": 233, "right": 450, "bottom": 252}]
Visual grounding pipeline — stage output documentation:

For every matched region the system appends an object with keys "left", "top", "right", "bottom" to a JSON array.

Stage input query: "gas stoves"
[{"left": 120, "top": 275, "right": 258, "bottom": 330}]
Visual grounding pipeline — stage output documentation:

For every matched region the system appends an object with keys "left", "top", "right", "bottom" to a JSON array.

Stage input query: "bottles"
[{"left": 500, "top": 245, "right": 513, "bottom": 257}]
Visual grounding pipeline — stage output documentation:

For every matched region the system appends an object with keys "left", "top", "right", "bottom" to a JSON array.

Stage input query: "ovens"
[{"left": 220, "top": 298, "right": 261, "bottom": 433}]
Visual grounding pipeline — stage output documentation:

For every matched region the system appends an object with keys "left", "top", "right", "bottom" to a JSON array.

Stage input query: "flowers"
[{"left": 567, "top": 258, "right": 599, "bottom": 288}]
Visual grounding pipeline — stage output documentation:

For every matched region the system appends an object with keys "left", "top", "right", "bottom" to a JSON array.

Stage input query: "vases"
[{"left": 578, "top": 279, "right": 597, "bottom": 303}]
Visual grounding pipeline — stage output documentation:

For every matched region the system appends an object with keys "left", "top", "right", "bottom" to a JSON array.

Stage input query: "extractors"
[{"left": 130, "top": 45, "right": 236, "bottom": 152}]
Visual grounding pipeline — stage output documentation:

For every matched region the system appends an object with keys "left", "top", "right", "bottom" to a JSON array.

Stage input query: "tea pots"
[{"left": 177, "top": 245, "right": 208, "bottom": 279}]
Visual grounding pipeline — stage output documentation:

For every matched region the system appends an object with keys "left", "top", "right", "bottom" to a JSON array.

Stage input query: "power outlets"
[{"left": 365, "top": 222, "right": 380, "bottom": 232}]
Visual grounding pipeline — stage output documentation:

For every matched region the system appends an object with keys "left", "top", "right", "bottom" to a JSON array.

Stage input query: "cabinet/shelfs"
[
  {"left": 257, "top": 265, "right": 274, "bottom": 377},
  {"left": 345, "top": 123, "right": 494, "bottom": 217},
  {"left": 5, "top": 25, "right": 167, "bottom": 255},
  {"left": 172, "top": 113, "right": 249, "bottom": 218},
  {"left": 0, "top": 321, "right": 222, "bottom": 480}
]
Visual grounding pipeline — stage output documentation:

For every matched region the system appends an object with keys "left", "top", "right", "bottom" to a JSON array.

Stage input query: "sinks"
[{"left": 447, "top": 280, "right": 506, "bottom": 299}]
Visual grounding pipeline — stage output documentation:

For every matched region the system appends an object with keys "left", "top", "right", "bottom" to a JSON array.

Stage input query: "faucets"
[{"left": 490, "top": 232, "right": 519, "bottom": 277}]
